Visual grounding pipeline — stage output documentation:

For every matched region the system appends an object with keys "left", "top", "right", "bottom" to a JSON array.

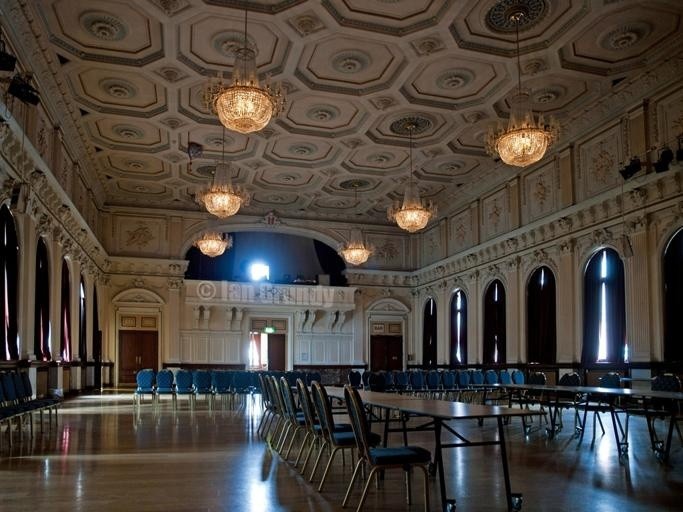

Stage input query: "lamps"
[
  {"left": 484, "top": 10, "right": 562, "bottom": 171},
  {"left": 202, "top": 0, "right": 286, "bottom": 136},
  {"left": 335, "top": 183, "right": 376, "bottom": 266},
  {"left": 189, "top": 228, "right": 236, "bottom": 259},
  {"left": 385, "top": 120, "right": 440, "bottom": 236},
  {"left": 197, "top": 125, "right": 250, "bottom": 222}
]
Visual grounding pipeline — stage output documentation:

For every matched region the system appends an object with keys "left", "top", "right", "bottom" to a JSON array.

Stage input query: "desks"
[
  {"left": 368, "top": 399, "right": 548, "bottom": 511},
  {"left": 291, "top": 385, "right": 428, "bottom": 477}
]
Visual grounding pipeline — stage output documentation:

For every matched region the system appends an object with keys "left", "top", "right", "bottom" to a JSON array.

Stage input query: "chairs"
[
  {"left": 253, "top": 368, "right": 526, "bottom": 410},
  {"left": 341, "top": 380, "right": 431, "bottom": 512},
  {"left": 131, "top": 367, "right": 250, "bottom": 413},
  {"left": 0, "top": 367, "right": 62, "bottom": 447},
  {"left": 473, "top": 368, "right": 682, "bottom": 461},
  {"left": 309, "top": 380, "right": 382, "bottom": 491},
  {"left": 258, "top": 373, "right": 356, "bottom": 472}
]
